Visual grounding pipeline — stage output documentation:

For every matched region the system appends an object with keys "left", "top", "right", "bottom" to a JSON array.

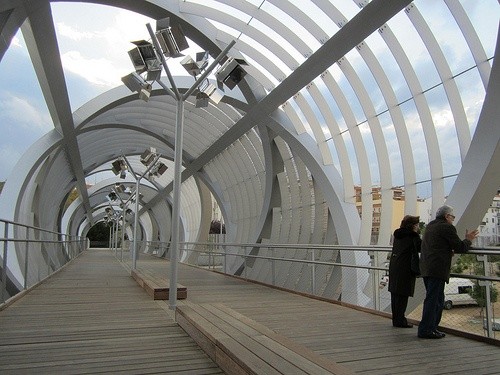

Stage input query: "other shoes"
[
  {"left": 433, "top": 328, "right": 446, "bottom": 337},
  {"left": 417, "top": 332, "right": 442, "bottom": 338},
  {"left": 393, "top": 323, "right": 413, "bottom": 328}
]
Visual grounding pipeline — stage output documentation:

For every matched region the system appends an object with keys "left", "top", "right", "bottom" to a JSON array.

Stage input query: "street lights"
[
  {"left": 103, "top": 184, "right": 144, "bottom": 262},
  {"left": 111, "top": 146, "right": 168, "bottom": 270},
  {"left": 122, "top": 17, "right": 250, "bottom": 310}
]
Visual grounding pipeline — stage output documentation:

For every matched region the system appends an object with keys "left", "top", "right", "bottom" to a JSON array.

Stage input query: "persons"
[
  {"left": 388, "top": 215, "right": 423, "bottom": 327},
  {"left": 417, "top": 206, "right": 479, "bottom": 338}
]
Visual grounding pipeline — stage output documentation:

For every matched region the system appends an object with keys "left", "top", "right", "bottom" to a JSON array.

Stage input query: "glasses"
[{"left": 449, "top": 214, "right": 455, "bottom": 219}]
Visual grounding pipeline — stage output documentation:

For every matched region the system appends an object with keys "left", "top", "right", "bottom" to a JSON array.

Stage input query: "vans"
[{"left": 444, "top": 278, "right": 478, "bottom": 310}]
